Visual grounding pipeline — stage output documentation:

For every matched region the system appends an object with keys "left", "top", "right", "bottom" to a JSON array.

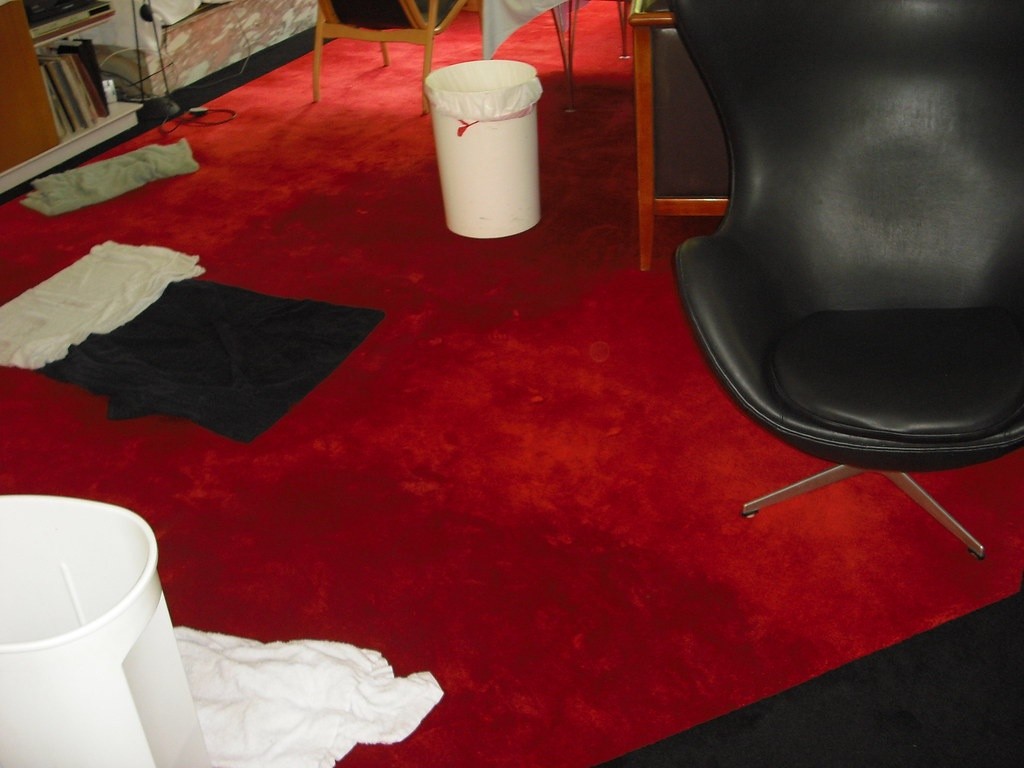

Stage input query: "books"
[{"left": 29, "top": 0, "right": 112, "bottom": 140}]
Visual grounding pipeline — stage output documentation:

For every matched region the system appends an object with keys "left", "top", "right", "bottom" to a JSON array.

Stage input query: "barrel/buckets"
[{"left": 0, "top": 495, "right": 211, "bottom": 768}]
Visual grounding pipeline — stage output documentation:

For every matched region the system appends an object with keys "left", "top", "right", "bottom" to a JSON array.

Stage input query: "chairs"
[
  {"left": 311, "top": 0, "right": 468, "bottom": 116},
  {"left": 665, "top": 0, "right": 1024, "bottom": 562}
]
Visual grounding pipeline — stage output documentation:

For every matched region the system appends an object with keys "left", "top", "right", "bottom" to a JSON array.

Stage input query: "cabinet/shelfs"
[{"left": 0, "top": 0, "right": 147, "bottom": 206}]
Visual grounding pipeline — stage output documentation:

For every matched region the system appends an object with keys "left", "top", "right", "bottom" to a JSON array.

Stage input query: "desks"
[{"left": 480, "top": 0, "right": 635, "bottom": 113}]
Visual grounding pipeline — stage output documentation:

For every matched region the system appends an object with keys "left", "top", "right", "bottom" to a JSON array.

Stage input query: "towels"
[
  {"left": 18, "top": 137, "right": 199, "bottom": 218},
  {"left": 171, "top": 624, "right": 445, "bottom": 768},
  {"left": 0, "top": 239, "right": 208, "bottom": 371},
  {"left": 35, "top": 278, "right": 386, "bottom": 445}
]
